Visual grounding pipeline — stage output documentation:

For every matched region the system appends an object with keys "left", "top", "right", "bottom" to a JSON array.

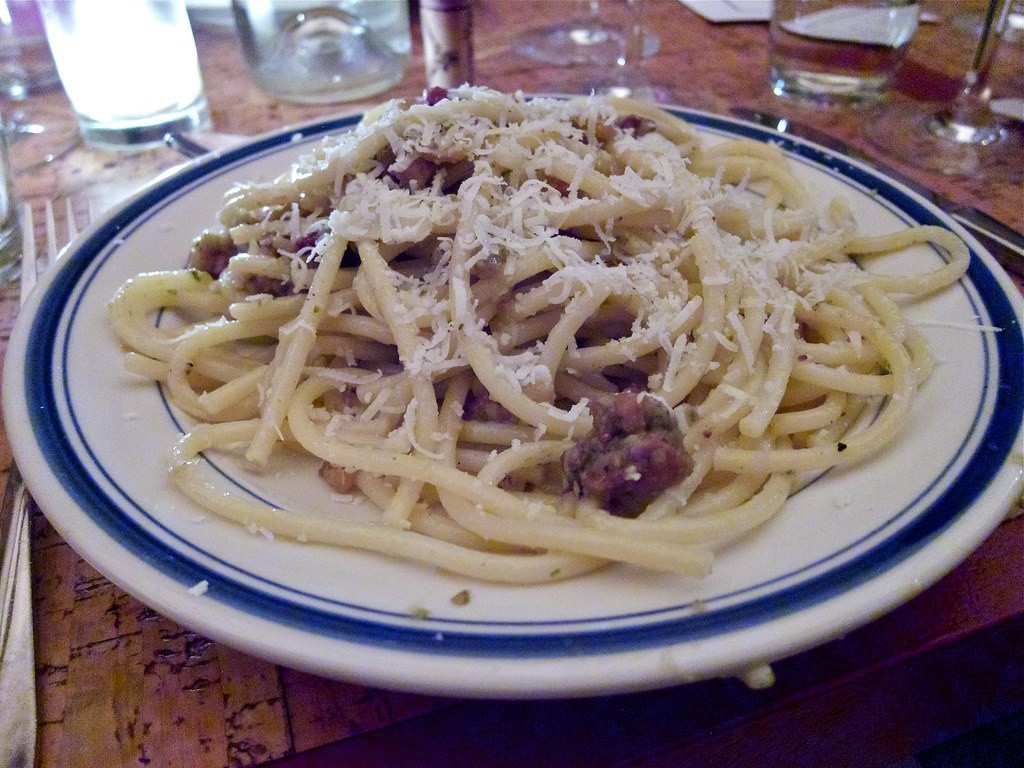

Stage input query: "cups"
[
  {"left": 767, "top": 1, "right": 921, "bottom": 115},
  {"left": 229, "top": 0, "right": 409, "bottom": 102},
  {"left": 37, "top": 0, "right": 211, "bottom": 152},
  {"left": 1, "top": 0, "right": 65, "bottom": 94},
  {"left": 1, "top": 133, "right": 21, "bottom": 276}
]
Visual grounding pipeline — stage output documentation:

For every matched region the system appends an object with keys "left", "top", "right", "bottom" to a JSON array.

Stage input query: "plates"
[{"left": 0, "top": 94, "right": 1024, "bottom": 702}]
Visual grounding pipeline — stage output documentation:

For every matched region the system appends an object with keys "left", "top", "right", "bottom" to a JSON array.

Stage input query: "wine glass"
[
  {"left": 1, "top": 67, "right": 81, "bottom": 171},
  {"left": 514, "top": 0, "right": 663, "bottom": 71},
  {"left": 861, "top": 0, "right": 1024, "bottom": 181},
  {"left": 572, "top": 1, "right": 692, "bottom": 103}
]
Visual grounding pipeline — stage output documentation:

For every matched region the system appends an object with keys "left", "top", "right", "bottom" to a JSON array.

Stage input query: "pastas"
[{"left": 109, "top": 80, "right": 971, "bottom": 585}]
